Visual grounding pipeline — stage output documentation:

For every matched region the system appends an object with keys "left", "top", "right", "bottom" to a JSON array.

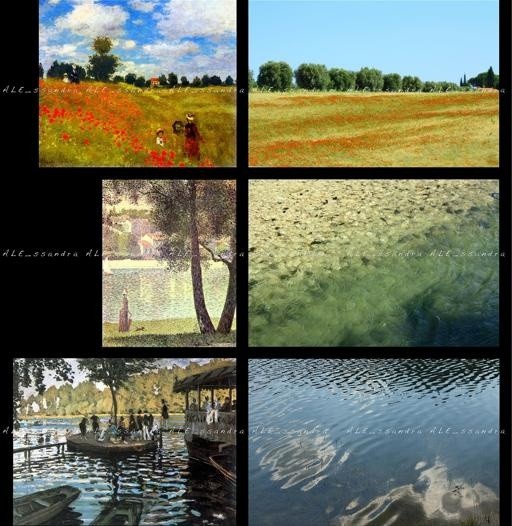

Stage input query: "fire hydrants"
[{"left": 119, "top": 288, "right": 129, "bottom": 332}]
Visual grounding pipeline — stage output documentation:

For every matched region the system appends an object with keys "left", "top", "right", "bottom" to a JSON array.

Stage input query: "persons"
[
  {"left": 24, "top": 429, "right": 71, "bottom": 444},
  {"left": 71, "top": 73, "right": 80, "bottom": 85},
  {"left": 156, "top": 127, "right": 166, "bottom": 147},
  {"left": 78, "top": 408, "right": 154, "bottom": 444},
  {"left": 190, "top": 394, "right": 236, "bottom": 413},
  {"left": 118, "top": 287, "right": 132, "bottom": 332},
  {"left": 183, "top": 112, "right": 206, "bottom": 162},
  {"left": 62, "top": 73, "right": 70, "bottom": 83},
  {"left": 159, "top": 398, "right": 169, "bottom": 428}
]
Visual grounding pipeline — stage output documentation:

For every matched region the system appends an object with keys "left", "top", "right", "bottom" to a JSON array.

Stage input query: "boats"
[
  {"left": 209, "top": 454, "right": 237, "bottom": 487},
  {"left": 12, "top": 485, "right": 81, "bottom": 526},
  {"left": 88, "top": 498, "right": 143, "bottom": 526}
]
